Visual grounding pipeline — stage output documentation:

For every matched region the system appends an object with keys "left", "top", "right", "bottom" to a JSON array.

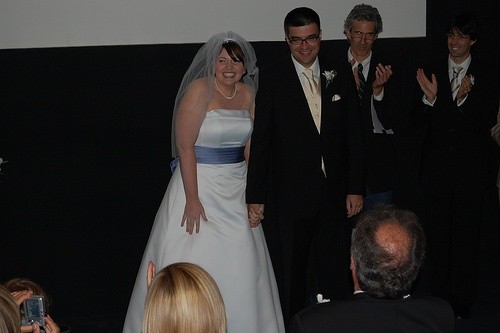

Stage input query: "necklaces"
[{"left": 214, "top": 77, "right": 237, "bottom": 99}]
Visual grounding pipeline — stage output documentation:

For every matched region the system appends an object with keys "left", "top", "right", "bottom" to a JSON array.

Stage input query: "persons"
[
  {"left": 334, "top": 4, "right": 415, "bottom": 206},
  {"left": 413, "top": 11, "right": 500, "bottom": 333},
  {"left": 0, "top": 279, "right": 60, "bottom": 333},
  {"left": 287, "top": 202, "right": 456, "bottom": 333},
  {"left": 245, "top": 7, "right": 366, "bottom": 323},
  {"left": 174, "top": 30, "right": 266, "bottom": 333},
  {"left": 141, "top": 260, "right": 227, "bottom": 333}
]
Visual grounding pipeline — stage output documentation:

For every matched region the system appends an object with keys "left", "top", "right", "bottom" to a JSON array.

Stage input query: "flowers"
[
  {"left": 322, "top": 69, "right": 338, "bottom": 87},
  {"left": 470, "top": 74, "right": 476, "bottom": 85},
  {"left": 315, "top": 294, "right": 330, "bottom": 304}
]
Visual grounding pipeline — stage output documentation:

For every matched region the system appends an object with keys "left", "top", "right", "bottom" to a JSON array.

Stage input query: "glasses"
[
  {"left": 446, "top": 32, "right": 470, "bottom": 41},
  {"left": 349, "top": 30, "right": 376, "bottom": 41},
  {"left": 287, "top": 32, "right": 319, "bottom": 45}
]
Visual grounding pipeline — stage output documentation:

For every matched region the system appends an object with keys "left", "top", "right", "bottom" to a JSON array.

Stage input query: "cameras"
[{"left": 23, "top": 294, "right": 46, "bottom": 327}]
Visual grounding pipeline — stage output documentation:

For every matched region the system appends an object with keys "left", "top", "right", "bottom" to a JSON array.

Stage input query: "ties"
[
  {"left": 357, "top": 63, "right": 366, "bottom": 106},
  {"left": 450, "top": 65, "right": 464, "bottom": 101},
  {"left": 302, "top": 69, "right": 317, "bottom": 96}
]
